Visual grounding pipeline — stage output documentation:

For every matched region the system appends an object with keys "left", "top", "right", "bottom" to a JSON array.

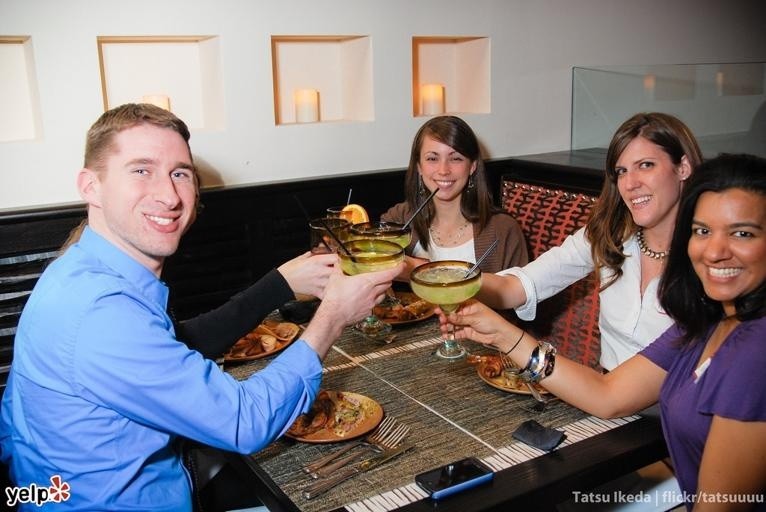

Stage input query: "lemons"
[{"left": 339, "top": 204, "right": 369, "bottom": 225}]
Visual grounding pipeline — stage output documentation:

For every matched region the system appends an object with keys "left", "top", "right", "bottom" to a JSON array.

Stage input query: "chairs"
[{"left": 498, "top": 178, "right": 613, "bottom": 378}]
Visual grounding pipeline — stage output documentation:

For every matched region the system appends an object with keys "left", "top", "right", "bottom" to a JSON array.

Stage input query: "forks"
[
  {"left": 302, "top": 416, "right": 398, "bottom": 474},
  {"left": 499, "top": 350, "right": 545, "bottom": 402},
  {"left": 310, "top": 423, "right": 411, "bottom": 479}
]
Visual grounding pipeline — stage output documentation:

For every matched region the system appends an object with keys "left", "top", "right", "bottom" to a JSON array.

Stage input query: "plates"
[
  {"left": 372, "top": 293, "right": 439, "bottom": 324},
  {"left": 284, "top": 390, "right": 383, "bottom": 442},
  {"left": 477, "top": 352, "right": 550, "bottom": 394},
  {"left": 224, "top": 320, "right": 299, "bottom": 360}
]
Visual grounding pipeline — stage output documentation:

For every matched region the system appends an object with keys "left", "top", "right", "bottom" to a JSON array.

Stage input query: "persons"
[
  {"left": 433, "top": 155, "right": 766, "bottom": 512},
  {"left": 369, "top": 116, "right": 529, "bottom": 338},
  {"left": 472, "top": 114, "right": 703, "bottom": 377},
  {"left": 62, "top": 166, "right": 340, "bottom": 362},
  {"left": 0, "top": 103, "right": 407, "bottom": 512}
]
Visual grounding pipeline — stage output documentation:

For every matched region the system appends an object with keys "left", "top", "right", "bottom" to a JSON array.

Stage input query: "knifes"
[{"left": 301, "top": 442, "right": 417, "bottom": 500}]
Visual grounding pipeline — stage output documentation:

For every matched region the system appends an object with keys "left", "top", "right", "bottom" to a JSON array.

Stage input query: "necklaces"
[
  {"left": 428, "top": 221, "right": 470, "bottom": 247},
  {"left": 634, "top": 224, "right": 675, "bottom": 258}
]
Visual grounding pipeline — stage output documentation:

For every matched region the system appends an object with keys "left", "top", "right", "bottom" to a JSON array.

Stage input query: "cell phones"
[{"left": 415, "top": 456, "right": 495, "bottom": 498}]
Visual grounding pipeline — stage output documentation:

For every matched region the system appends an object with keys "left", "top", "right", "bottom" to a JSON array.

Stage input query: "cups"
[
  {"left": 350, "top": 221, "right": 413, "bottom": 249},
  {"left": 410, "top": 260, "right": 482, "bottom": 359},
  {"left": 309, "top": 218, "right": 350, "bottom": 254},
  {"left": 326, "top": 206, "right": 353, "bottom": 224},
  {"left": 337, "top": 239, "right": 404, "bottom": 338}
]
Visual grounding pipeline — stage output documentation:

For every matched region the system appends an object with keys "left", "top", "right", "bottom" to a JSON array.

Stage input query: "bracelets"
[{"left": 516, "top": 342, "right": 556, "bottom": 381}]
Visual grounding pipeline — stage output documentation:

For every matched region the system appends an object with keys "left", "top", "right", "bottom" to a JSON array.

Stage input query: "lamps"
[
  {"left": 295, "top": 89, "right": 319, "bottom": 123},
  {"left": 144, "top": 95, "right": 170, "bottom": 109},
  {"left": 422, "top": 83, "right": 445, "bottom": 116}
]
